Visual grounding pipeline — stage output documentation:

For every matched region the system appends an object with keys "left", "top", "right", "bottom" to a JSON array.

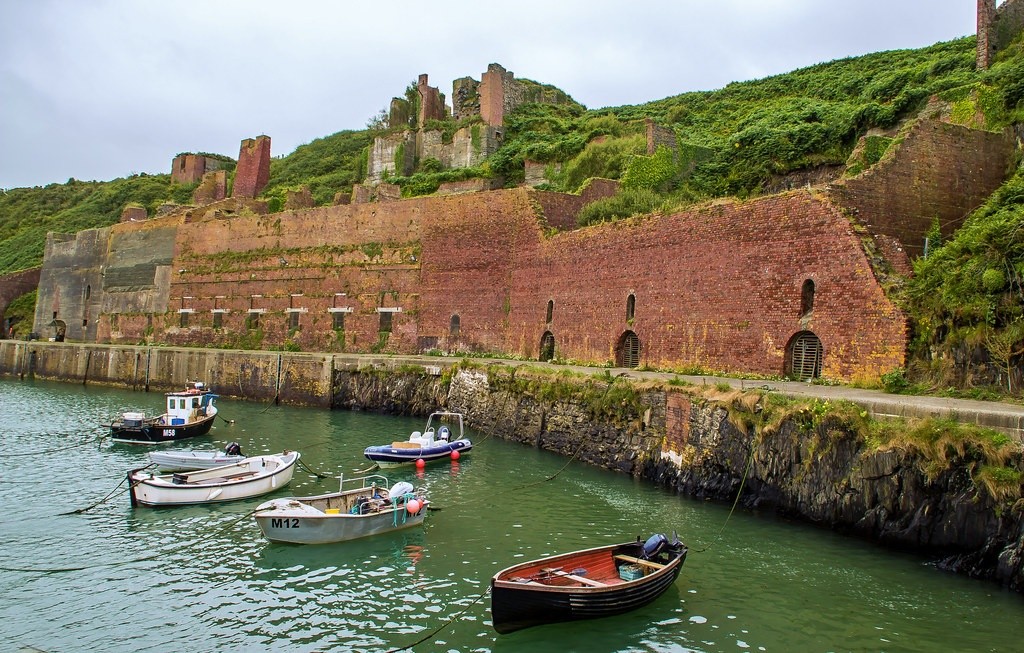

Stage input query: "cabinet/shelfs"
[{"left": 221, "top": 471, "right": 258, "bottom": 482}]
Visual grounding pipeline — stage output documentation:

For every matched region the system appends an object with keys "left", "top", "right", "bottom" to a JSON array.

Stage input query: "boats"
[
  {"left": 490, "top": 532, "right": 688, "bottom": 633},
  {"left": 364, "top": 411, "right": 474, "bottom": 469},
  {"left": 129, "top": 443, "right": 303, "bottom": 505},
  {"left": 257, "top": 476, "right": 443, "bottom": 544},
  {"left": 101, "top": 373, "right": 217, "bottom": 441}
]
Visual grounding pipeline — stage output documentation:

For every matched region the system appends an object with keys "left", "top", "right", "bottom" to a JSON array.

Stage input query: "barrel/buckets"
[{"left": 325, "top": 509, "right": 340, "bottom": 514}]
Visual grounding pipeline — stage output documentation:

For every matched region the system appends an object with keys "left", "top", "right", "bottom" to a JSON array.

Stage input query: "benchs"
[
  {"left": 540, "top": 567, "right": 608, "bottom": 587},
  {"left": 613, "top": 555, "right": 665, "bottom": 570}
]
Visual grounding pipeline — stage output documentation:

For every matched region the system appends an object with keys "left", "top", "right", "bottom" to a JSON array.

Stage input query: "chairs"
[
  {"left": 409, "top": 431, "right": 421, "bottom": 444},
  {"left": 420, "top": 431, "right": 435, "bottom": 446},
  {"left": 437, "top": 427, "right": 449, "bottom": 445}
]
